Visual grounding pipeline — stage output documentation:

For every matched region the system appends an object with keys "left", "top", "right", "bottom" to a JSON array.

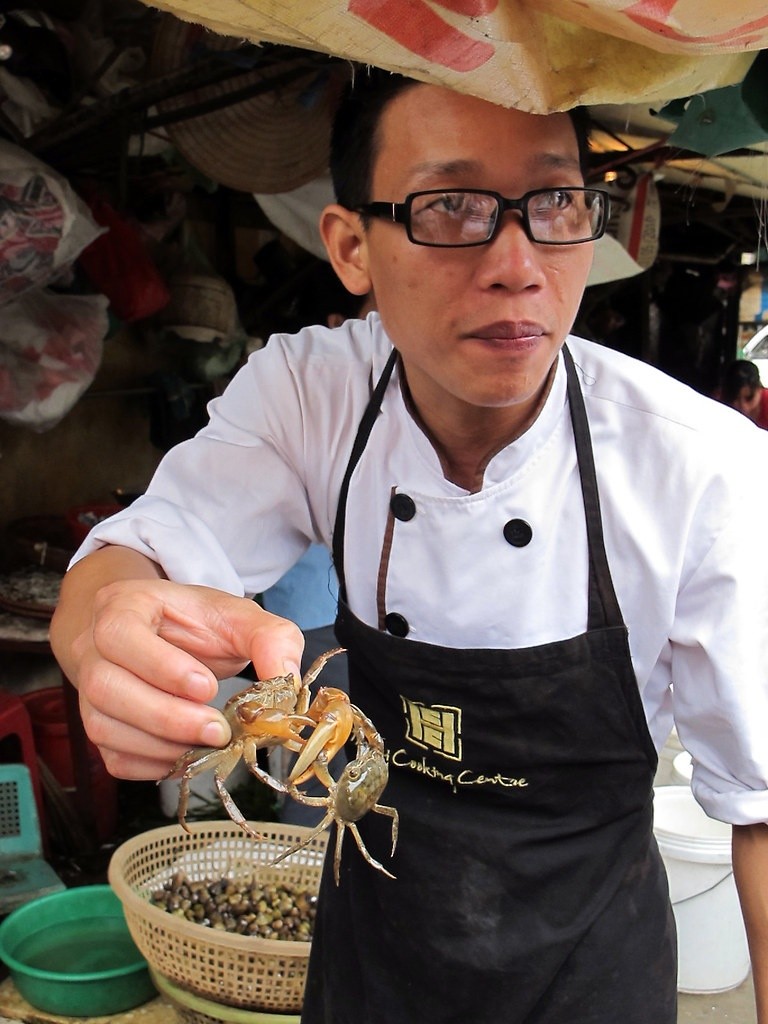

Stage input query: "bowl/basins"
[{"left": 0, "top": 879, "right": 160, "bottom": 1016}]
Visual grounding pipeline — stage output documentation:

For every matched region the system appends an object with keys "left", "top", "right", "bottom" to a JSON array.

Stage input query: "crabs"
[{"left": 154, "top": 647, "right": 399, "bottom": 887}]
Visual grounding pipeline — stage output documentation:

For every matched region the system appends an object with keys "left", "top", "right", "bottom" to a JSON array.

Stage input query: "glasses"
[{"left": 353, "top": 185, "right": 613, "bottom": 249}]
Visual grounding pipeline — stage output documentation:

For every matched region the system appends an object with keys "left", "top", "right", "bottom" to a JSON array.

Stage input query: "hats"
[{"left": 152, "top": 14, "right": 355, "bottom": 195}]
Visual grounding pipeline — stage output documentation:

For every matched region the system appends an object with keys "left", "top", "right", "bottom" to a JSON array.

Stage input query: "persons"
[
  {"left": 710, "top": 360, "right": 768, "bottom": 429},
  {"left": 50, "top": 78, "right": 768, "bottom": 1024}
]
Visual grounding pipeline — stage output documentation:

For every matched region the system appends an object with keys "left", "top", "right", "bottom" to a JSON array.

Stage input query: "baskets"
[{"left": 108, "top": 821, "right": 333, "bottom": 1014}]
